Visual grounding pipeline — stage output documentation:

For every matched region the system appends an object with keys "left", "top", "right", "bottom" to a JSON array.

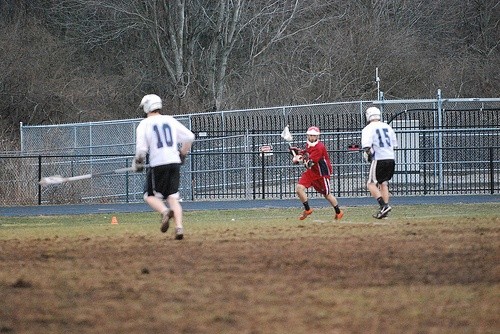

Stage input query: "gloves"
[
  {"left": 289, "top": 146, "right": 303, "bottom": 156},
  {"left": 178, "top": 151, "right": 187, "bottom": 166},
  {"left": 132, "top": 157, "right": 145, "bottom": 173},
  {"left": 292, "top": 154, "right": 305, "bottom": 164},
  {"left": 364, "top": 151, "right": 374, "bottom": 165}
]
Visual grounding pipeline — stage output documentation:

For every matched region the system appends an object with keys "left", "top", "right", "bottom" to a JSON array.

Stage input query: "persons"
[
  {"left": 290, "top": 126, "right": 344, "bottom": 220},
  {"left": 362, "top": 107, "right": 398, "bottom": 219},
  {"left": 132, "top": 94, "right": 195, "bottom": 240}
]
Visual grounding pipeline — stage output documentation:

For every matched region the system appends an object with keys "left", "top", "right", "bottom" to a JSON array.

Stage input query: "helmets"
[
  {"left": 139, "top": 93, "right": 163, "bottom": 114},
  {"left": 364, "top": 107, "right": 381, "bottom": 123},
  {"left": 306, "top": 127, "right": 320, "bottom": 147}
]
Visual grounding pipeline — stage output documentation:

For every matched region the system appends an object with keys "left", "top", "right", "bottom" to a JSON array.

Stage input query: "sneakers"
[
  {"left": 299, "top": 207, "right": 313, "bottom": 220},
  {"left": 372, "top": 204, "right": 392, "bottom": 219},
  {"left": 160, "top": 208, "right": 174, "bottom": 233},
  {"left": 334, "top": 209, "right": 344, "bottom": 221},
  {"left": 172, "top": 227, "right": 184, "bottom": 240}
]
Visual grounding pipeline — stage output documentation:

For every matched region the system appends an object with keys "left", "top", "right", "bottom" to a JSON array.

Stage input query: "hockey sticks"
[
  {"left": 281, "top": 125, "right": 297, "bottom": 159},
  {"left": 39, "top": 164, "right": 150, "bottom": 188}
]
